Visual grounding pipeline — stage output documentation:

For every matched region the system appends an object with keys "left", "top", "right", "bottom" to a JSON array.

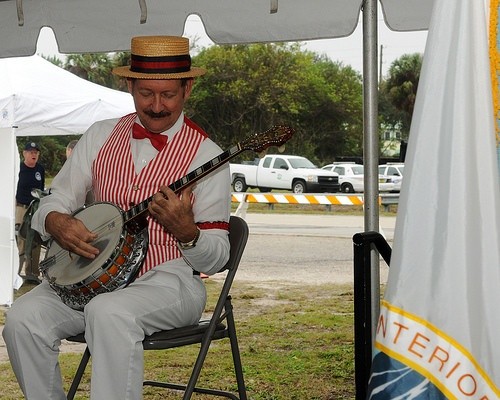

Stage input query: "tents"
[{"left": 0, "top": 53, "right": 138, "bottom": 309}]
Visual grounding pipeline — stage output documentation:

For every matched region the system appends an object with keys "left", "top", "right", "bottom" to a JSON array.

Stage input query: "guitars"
[{"left": 39, "top": 123, "right": 296, "bottom": 312}]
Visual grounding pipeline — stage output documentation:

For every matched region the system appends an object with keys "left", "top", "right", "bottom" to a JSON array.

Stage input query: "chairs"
[{"left": 64, "top": 215, "right": 251, "bottom": 399}]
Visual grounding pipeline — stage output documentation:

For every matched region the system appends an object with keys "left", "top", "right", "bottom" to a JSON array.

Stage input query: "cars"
[
  {"left": 379, "top": 163, "right": 404, "bottom": 191},
  {"left": 322, "top": 161, "right": 394, "bottom": 194}
]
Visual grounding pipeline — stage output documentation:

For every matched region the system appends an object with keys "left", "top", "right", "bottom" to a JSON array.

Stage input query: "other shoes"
[{"left": 25, "top": 278, "right": 43, "bottom": 285}]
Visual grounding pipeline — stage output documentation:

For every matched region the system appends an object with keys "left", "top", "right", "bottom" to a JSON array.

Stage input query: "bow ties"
[{"left": 132, "top": 122, "right": 168, "bottom": 152}]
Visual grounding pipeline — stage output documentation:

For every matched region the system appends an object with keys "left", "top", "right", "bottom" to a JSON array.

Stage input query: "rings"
[{"left": 71, "top": 245, "right": 77, "bottom": 251}]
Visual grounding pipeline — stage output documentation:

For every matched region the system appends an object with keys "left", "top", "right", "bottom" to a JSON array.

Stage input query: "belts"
[{"left": 17, "top": 202, "right": 29, "bottom": 209}]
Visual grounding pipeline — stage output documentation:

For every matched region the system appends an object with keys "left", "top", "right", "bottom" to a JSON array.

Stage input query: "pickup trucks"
[{"left": 229, "top": 154, "right": 342, "bottom": 194}]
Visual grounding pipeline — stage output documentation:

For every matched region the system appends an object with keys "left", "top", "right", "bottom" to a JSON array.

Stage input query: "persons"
[
  {"left": 16, "top": 141, "right": 45, "bottom": 283},
  {"left": 5, "top": 35, "right": 231, "bottom": 400},
  {"left": 64, "top": 140, "right": 80, "bottom": 159}
]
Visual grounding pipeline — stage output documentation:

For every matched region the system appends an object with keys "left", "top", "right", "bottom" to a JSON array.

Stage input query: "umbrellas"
[{"left": 0, "top": 0, "right": 500, "bottom": 400}]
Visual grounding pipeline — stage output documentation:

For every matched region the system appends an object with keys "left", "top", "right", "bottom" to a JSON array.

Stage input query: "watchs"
[{"left": 178, "top": 226, "right": 200, "bottom": 249}]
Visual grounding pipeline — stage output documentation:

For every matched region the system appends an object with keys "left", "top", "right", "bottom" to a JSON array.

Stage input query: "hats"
[
  {"left": 26, "top": 141, "right": 41, "bottom": 152},
  {"left": 111, "top": 36, "right": 207, "bottom": 79}
]
